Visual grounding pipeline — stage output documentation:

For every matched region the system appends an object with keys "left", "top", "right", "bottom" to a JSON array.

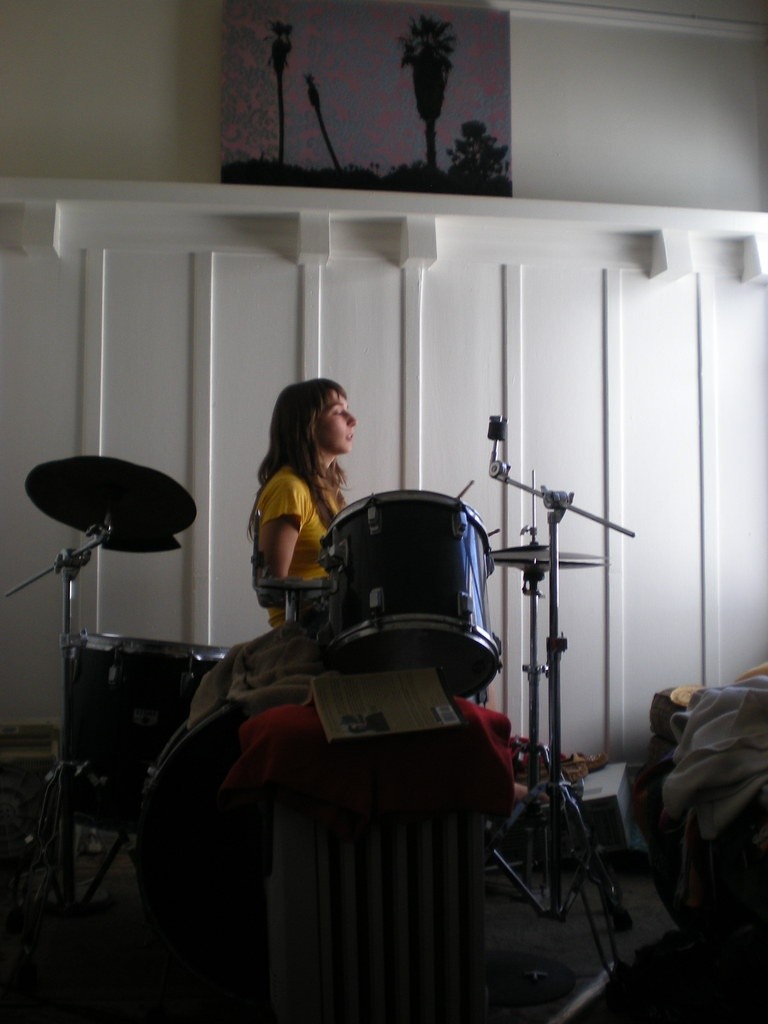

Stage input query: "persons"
[{"left": 247, "top": 380, "right": 551, "bottom": 803}]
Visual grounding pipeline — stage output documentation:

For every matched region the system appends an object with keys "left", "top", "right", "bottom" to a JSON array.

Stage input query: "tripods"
[
  {"left": 470, "top": 416, "right": 634, "bottom": 979},
  {"left": 0, "top": 509, "right": 142, "bottom": 1000}
]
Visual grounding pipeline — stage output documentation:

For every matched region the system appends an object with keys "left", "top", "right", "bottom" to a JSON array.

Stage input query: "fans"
[{"left": 0, "top": 724, "right": 61, "bottom": 862}]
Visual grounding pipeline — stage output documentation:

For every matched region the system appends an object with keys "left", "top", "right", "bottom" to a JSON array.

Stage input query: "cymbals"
[
  {"left": 487, "top": 540, "right": 606, "bottom": 575},
  {"left": 23, "top": 452, "right": 199, "bottom": 544}
]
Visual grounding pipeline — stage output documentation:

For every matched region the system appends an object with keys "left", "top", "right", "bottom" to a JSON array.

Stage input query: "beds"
[{"left": 638, "top": 686, "right": 767, "bottom": 944}]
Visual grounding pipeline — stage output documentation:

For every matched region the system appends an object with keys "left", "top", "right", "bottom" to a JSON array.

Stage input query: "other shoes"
[
  {"left": 516, "top": 761, "right": 589, "bottom": 786},
  {"left": 574, "top": 751, "right": 609, "bottom": 770}
]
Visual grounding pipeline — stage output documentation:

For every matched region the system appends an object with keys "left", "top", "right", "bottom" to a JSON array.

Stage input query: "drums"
[
  {"left": 132, "top": 697, "right": 269, "bottom": 1010},
  {"left": 322, "top": 487, "right": 502, "bottom": 700},
  {"left": 57, "top": 630, "right": 235, "bottom": 833}
]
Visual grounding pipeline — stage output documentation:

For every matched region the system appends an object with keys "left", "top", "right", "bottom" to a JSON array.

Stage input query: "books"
[{"left": 302, "top": 667, "right": 468, "bottom": 745}]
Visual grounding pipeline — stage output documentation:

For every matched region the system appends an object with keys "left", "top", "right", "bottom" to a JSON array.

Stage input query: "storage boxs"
[{"left": 540, "top": 761, "right": 635, "bottom": 855}]
